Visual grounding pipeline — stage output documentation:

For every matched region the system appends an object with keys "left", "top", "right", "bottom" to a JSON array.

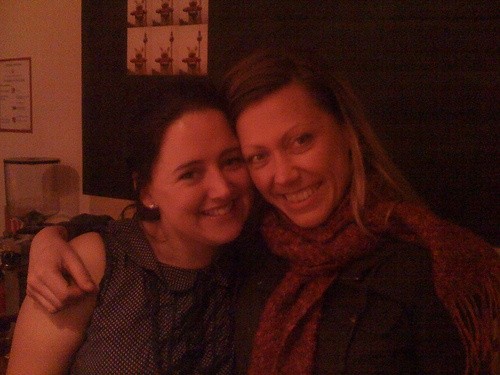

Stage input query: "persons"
[
  {"left": 5, "top": 77, "right": 291, "bottom": 374},
  {"left": 24, "top": 53, "right": 500, "bottom": 375}
]
